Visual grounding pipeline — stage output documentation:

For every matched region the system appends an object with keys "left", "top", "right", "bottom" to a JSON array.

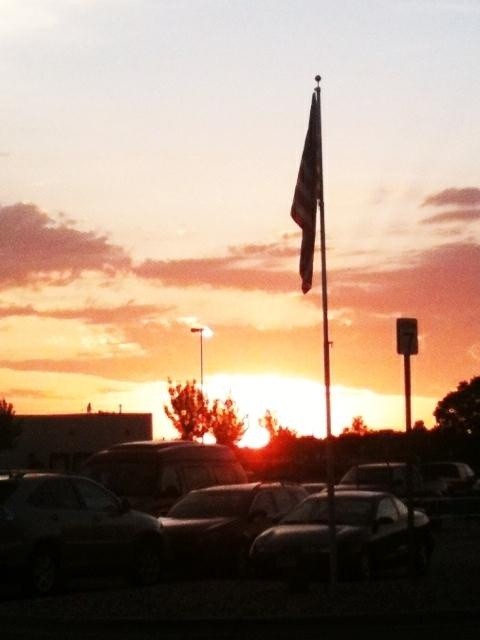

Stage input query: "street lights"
[{"left": 191, "top": 328, "right": 204, "bottom": 442}]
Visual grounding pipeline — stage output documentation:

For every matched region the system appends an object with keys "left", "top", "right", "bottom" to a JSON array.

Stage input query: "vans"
[{"left": 105, "top": 440, "right": 247, "bottom": 517}]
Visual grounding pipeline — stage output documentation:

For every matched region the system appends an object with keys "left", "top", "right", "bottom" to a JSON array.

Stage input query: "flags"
[{"left": 289, "top": 83, "right": 324, "bottom": 296}]
